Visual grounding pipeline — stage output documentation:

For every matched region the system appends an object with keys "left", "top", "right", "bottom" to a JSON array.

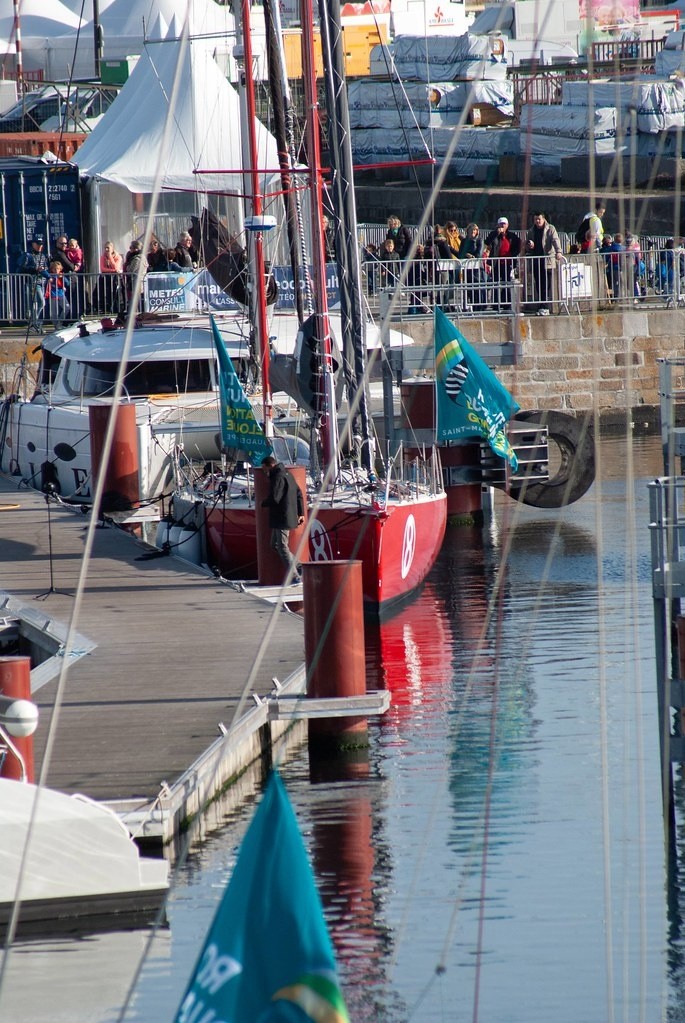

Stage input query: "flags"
[
  {"left": 209, "top": 313, "right": 273, "bottom": 468},
  {"left": 433, "top": 304, "right": 520, "bottom": 475}
]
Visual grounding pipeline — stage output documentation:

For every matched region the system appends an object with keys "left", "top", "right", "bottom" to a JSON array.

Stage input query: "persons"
[
  {"left": 320, "top": 202, "right": 685, "bottom": 318},
  {"left": 16, "top": 231, "right": 200, "bottom": 334},
  {"left": 260, "top": 456, "right": 304, "bottom": 585}
]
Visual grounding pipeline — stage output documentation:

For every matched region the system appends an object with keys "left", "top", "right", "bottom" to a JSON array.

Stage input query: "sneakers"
[{"left": 535, "top": 308, "right": 550, "bottom": 316}]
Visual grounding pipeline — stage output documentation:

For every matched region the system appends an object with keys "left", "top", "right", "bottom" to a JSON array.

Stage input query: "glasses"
[
  {"left": 56, "top": 242, "right": 68, "bottom": 246},
  {"left": 448, "top": 229, "right": 457, "bottom": 232}
]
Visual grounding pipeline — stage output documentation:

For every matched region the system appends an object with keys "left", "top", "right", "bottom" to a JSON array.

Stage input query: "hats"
[
  {"left": 664, "top": 239, "right": 674, "bottom": 248},
  {"left": 602, "top": 234, "right": 613, "bottom": 243},
  {"left": 31, "top": 232, "right": 46, "bottom": 244},
  {"left": 498, "top": 216, "right": 508, "bottom": 224}
]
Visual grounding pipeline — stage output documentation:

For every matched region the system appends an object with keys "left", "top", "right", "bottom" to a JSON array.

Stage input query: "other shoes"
[
  {"left": 289, "top": 577, "right": 303, "bottom": 587},
  {"left": 297, "top": 567, "right": 304, "bottom": 577},
  {"left": 25, "top": 325, "right": 48, "bottom": 335},
  {"left": 368, "top": 293, "right": 377, "bottom": 297},
  {"left": 633, "top": 298, "right": 641, "bottom": 304},
  {"left": 54, "top": 321, "right": 63, "bottom": 333}
]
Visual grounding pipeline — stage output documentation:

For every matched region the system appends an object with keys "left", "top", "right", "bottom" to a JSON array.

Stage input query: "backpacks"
[
  {"left": 575, "top": 215, "right": 595, "bottom": 243},
  {"left": 655, "top": 252, "right": 673, "bottom": 277},
  {"left": 608, "top": 249, "right": 622, "bottom": 264},
  {"left": 634, "top": 255, "right": 645, "bottom": 276},
  {"left": 423, "top": 240, "right": 446, "bottom": 269}
]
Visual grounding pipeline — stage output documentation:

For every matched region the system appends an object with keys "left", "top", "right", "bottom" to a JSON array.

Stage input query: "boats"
[{"left": 0, "top": 0, "right": 595, "bottom": 613}]
